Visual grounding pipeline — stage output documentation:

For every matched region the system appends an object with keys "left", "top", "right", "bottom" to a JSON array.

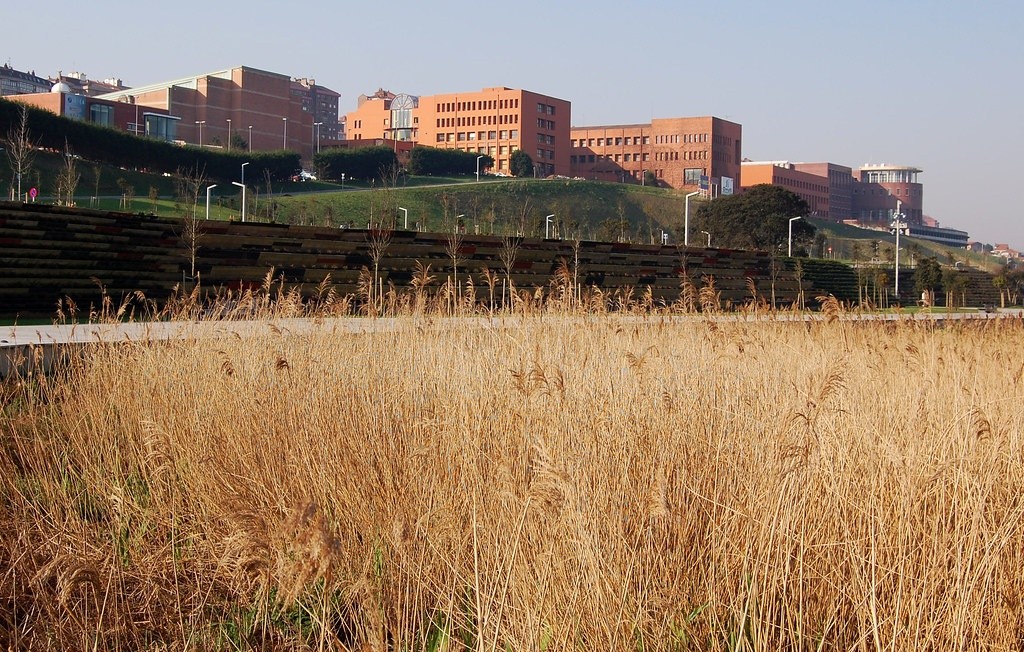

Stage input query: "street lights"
[
  {"left": 476, "top": 155, "right": 483, "bottom": 182},
  {"left": 242, "top": 162, "right": 250, "bottom": 224},
  {"left": 227, "top": 119, "right": 231, "bottom": 152},
  {"left": 314, "top": 122, "right": 323, "bottom": 154},
  {"left": 249, "top": 125, "right": 252, "bottom": 153},
  {"left": 196, "top": 121, "right": 206, "bottom": 148},
  {"left": 283, "top": 117, "right": 286, "bottom": 150},
  {"left": 206, "top": 184, "right": 217, "bottom": 220},
  {"left": 232, "top": 181, "right": 245, "bottom": 223},
  {"left": 399, "top": 207, "right": 408, "bottom": 230}
]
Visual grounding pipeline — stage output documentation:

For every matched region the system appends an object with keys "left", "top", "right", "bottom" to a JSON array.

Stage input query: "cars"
[{"left": 496, "top": 172, "right": 507, "bottom": 178}]
